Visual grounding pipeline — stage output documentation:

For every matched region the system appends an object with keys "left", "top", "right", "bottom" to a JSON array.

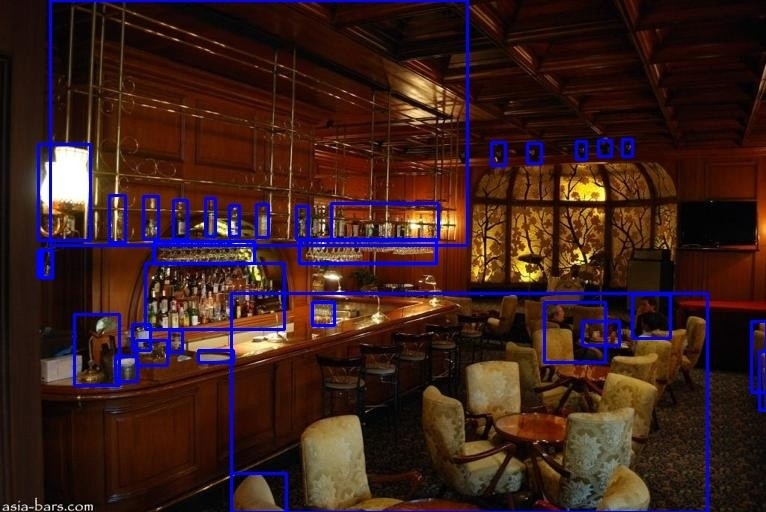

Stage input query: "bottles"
[
  {"left": 144, "top": 197, "right": 159, "bottom": 240},
  {"left": 173, "top": 200, "right": 187, "bottom": 239},
  {"left": 206, "top": 198, "right": 216, "bottom": 237},
  {"left": 137, "top": 340, "right": 167, "bottom": 365},
  {"left": 332, "top": 204, "right": 439, "bottom": 240},
  {"left": 109, "top": 196, "right": 125, "bottom": 242},
  {"left": 256, "top": 204, "right": 269, "bottom": 238},
  {"left": 296, "top": 206, "right": 309, "bottom": 240},
  {"left": 169, "top": 330, "right": 182, "bottom": 352},
  {"left": 133, "top": 325, "right": 150, "bottom": 350},
  {"left": 145, "top": 264, "right": 285, "bottom": 329},
  {"left": 583, "top": 322, "right": 619, "bottom": 345},
  {"left": 230, "top": 205, "right": 240, "bottom": 237}
]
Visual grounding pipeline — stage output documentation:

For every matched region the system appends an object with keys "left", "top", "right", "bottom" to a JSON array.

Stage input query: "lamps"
[
  {"left": 622, "top": 140, "right": 633, "bottom": 156},
  {"left": 577, "top": 142, "right": 587, "bottom": 160},
  {"left": 75, "top": 314, "right": 120, "bottom": 385},
  {"left": 38, "top": 145, "right": 90, "bottom": 240},
  {"left": 599, "top": 140, "right": 612, "bottom": 156},
  {"left": 491, "top": 142, "right": 505, "bottom": 166},
  {"left": 527, "top": 143, "right": 541, "bottom": 164}
]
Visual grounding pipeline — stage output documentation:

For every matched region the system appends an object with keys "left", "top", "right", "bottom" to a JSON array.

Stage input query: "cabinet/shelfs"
[{"left": 50, "top": 0, "right": 467, "bottom": 246}]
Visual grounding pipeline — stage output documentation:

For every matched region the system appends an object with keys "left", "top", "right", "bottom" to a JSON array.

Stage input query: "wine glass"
[
  {"left": 154, "top": 242, "right": 254, "bottom": 263},
  {"left": 39, "top": 251, "right": 42, "bottom": 278},
  {"left": 300, "top": 240, "right": 436, "bottom": 263},
  {"left": 312, "top": 302, "right": 334, "bottom": 326},
  {"left": 42, "top": 250, "right": 53, "bottom": 278}
]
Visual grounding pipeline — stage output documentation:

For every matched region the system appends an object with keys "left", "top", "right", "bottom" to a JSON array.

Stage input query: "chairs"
[
  {"left": 760, "top": 352, "right": 766, "bottom": 411},
  {"left": 231, "top": 473, "right": 287, "bottom": 512},
  {"left": 752, "top": 321, "right": 766, "bottom": 393},
  {"left": 232, "top": 293, "right": 707, "bottom": 512}
]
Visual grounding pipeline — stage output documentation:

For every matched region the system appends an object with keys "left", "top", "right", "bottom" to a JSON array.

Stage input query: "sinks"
[{"left": 198, "top": 351, "right": 234, "bottom": 363}]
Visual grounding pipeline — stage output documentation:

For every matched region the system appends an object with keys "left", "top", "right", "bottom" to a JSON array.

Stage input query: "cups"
[{"left": 115, "top": 357, "right": 137, "bottom": 382}]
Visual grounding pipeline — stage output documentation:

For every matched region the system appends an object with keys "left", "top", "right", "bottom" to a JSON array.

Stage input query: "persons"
[
  {"left": 633, "top": 295, "right": 670, "bottom": 338},
  {"left": 545, "top": 303, "right": 606, "bottom": 362}
]
